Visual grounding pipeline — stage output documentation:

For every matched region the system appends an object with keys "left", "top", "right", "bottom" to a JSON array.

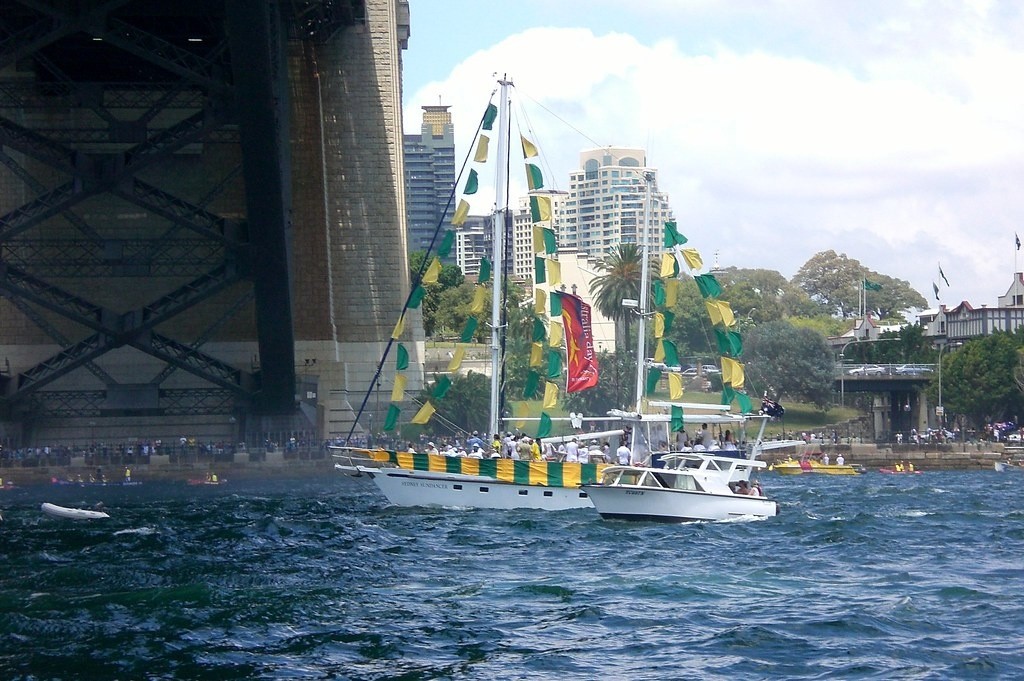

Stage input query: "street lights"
[
  {"left": 936, "top": 342, "right": 963, "bottom": 431},
  {"left": 839, "top": 338, "right": 902, "bottom": 408}
]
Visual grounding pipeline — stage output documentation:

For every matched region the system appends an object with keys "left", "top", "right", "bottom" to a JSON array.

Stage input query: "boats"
[
  {"left": 879, "top": 466, "right": 923, "bottom": 475},
  {"left": 42, "top": 502, "right": 111, "bottom": 527},
  {"left": 771, "top": 460, "right": 867, "bottom": 474},
  {"left": 51, "top": 476, "right": 145, "bottom": 487},
  {"left": 185, "top": 477, "right": 227, "bottom": 487},
  {"left": 579, "top": 170, "right": 780, "bottom": 521}
]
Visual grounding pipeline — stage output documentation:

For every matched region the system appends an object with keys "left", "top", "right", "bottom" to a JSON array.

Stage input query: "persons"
[
  {"left": 702, "top": 366, "right": 708, "bottom": 374},
  {"left": 644, "top": 360, "right": 664, "bottom": 372},
  {"left": 0, "top": 395, "right": 1024, "bottom": 496}
]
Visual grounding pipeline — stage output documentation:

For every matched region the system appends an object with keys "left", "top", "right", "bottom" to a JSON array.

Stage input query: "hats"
[
  {"left": 472, "top": 431, "right": 478, "bottom": 435},
  {"left": 838, "top": 453, "right": 842, "bottom": 457},
  {"left": 510, "top": 435, "right": 516, "bottom": 440},
  {"left": 591, "top": 439, "right": 597, "bottom": 444},
  {"left": 711, "top": 439, "right": 717, "bottom": 445},
  {"left": 507, "top": 431, "right": 512, "bottom": 436},
  {"left": 473, "top": 442, "right": 480, "bottom": 448},
  {"left": 428, "top": 441, "right": 435, "bottom": 447},
  {"left": 825, "top": 453, "right": 828, "bottom": 457},
  {"left": 458, "top": 447, "right": 464, "bottom": 450}
]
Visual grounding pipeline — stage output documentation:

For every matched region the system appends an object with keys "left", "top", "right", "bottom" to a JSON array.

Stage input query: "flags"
[
  {"left": 932, "top": 266, "right": 949, "bottom": 300},
  {"left": 1015, "top": 234, "right": 1021, "bottom": 250},
  {"left": 861, "top": 278, "right": 883, "bottom": 292},
  {"left": 761, "top": 396, "right": 784, "bottom": 422}
]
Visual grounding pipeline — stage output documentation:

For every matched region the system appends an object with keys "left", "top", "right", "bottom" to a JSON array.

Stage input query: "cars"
[
  {"left": 645, "top": 358, "right": 719, "bottom": 376},
  {"left": 911, "top": 430, "right": 956, "bottom": 444},
  {"left": 849, "top": 363, "right": 885, "bottom": 376},
  {"left": 896, "top": 363, "right": 933, "bottom": 375}
]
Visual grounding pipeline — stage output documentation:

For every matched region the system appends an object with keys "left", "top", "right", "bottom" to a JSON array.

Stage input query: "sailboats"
[{"left": 326, "top": 73, "right": 697, "bottom": 509}]
